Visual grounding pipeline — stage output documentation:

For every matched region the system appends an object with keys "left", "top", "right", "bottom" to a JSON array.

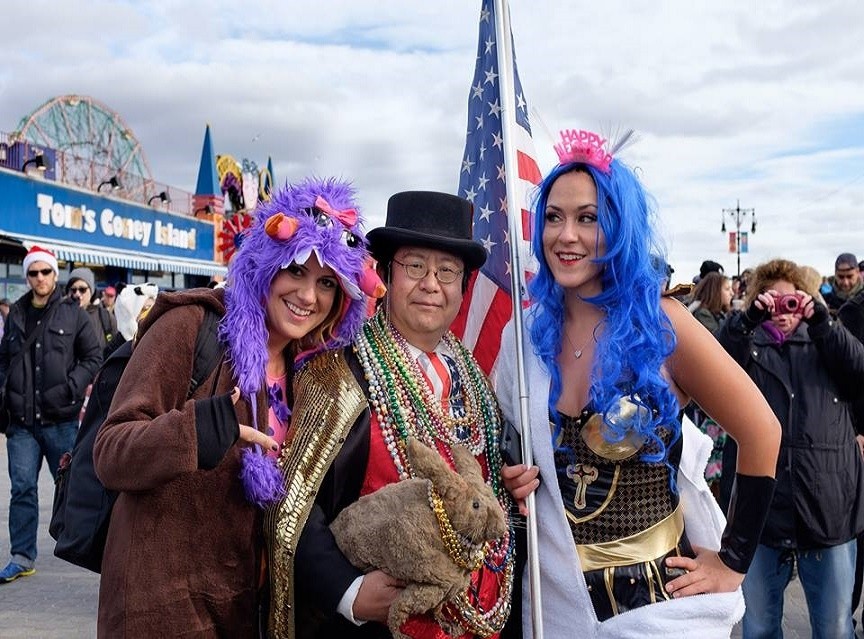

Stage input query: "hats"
[
  {"left": 835, "top": 253, "right": 858, "bottom": 272},
  {"left": 23, "top": 245, "right": 60, "bottom": 281},
  {"left": 699, "top": 260, "right": 725, "bottom": 279},
  {"left": 68, "top": 268, "right": 95, "bottom": 296},
  {"left": 105, "top": 286, "right": 116, "bottom": 296},
  {"left": 366, "top": 190, "right": 487, "bottom": 272}
]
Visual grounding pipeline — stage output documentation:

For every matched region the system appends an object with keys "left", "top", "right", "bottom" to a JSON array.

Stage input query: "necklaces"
[
  {"left": 574, "top": 350, "right": 582, "bottom": 358},
  {"left": 356, "top": 306, "right": 515, "bottom": 639}
]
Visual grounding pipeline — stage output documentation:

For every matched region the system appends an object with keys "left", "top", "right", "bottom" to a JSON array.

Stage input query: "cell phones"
[{"left": 500, "top": 421, "right": 521, "bottom": 466}]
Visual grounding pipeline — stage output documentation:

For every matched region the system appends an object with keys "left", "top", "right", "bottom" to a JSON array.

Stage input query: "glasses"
[
  {"left": 393, "top": 258, "right": 465, "bottom": 284},
  {"left": 71, "top": 287, "right": 89, "bottom": 294},
  {"left": 27, "top": 268, "right": 53, "bottom": 277}
]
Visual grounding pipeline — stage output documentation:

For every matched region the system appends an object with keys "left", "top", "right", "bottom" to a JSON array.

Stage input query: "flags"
[{"left": 449, "top": 0, "right": 543, "bottom": 377}]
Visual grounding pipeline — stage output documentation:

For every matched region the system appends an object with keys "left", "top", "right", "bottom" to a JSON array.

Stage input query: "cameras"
[{"left": 772, "top": 294, "right": 805, "bottom": 315}]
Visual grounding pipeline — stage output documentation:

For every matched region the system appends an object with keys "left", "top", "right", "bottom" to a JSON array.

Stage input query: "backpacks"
[{"left": 48, "top": 331, "right": 132, "bottom": 571}]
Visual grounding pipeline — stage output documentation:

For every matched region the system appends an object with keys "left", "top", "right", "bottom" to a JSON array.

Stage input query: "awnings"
[{"left": 22, "top": 239, "right": 229, "bottom": 277}]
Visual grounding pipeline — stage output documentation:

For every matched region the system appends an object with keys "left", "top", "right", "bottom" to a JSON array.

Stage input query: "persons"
[
  {"left": 264, "top": 190, "right": 527, "bottom": 639},
  {"left": 488, "top": 142, "right": 782, "bottom": 639},
  {"left": 93, "top": 181, "right": 374, "bottom": 639},
  {"left": 66, "top": 267, "right": 118, "bottom": 344},
  {"left": 0, "top": 246, "right": 104, "bottom": 584},
  {"left": 103, "top": 282, "right": 159, "bottom": 362},
  {"left": 689, "top": 254, "right": 864, "bottom": 639}
]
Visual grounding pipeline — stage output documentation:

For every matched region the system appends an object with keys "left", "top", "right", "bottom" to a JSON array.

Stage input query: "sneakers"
[{"left": 0, "top": 562, "right": 36, "bottom": 583}]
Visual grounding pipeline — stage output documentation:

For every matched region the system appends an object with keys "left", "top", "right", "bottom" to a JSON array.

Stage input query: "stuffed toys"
[{"left": 329, "top": 439, "right": 507, "bottom": 639}]
